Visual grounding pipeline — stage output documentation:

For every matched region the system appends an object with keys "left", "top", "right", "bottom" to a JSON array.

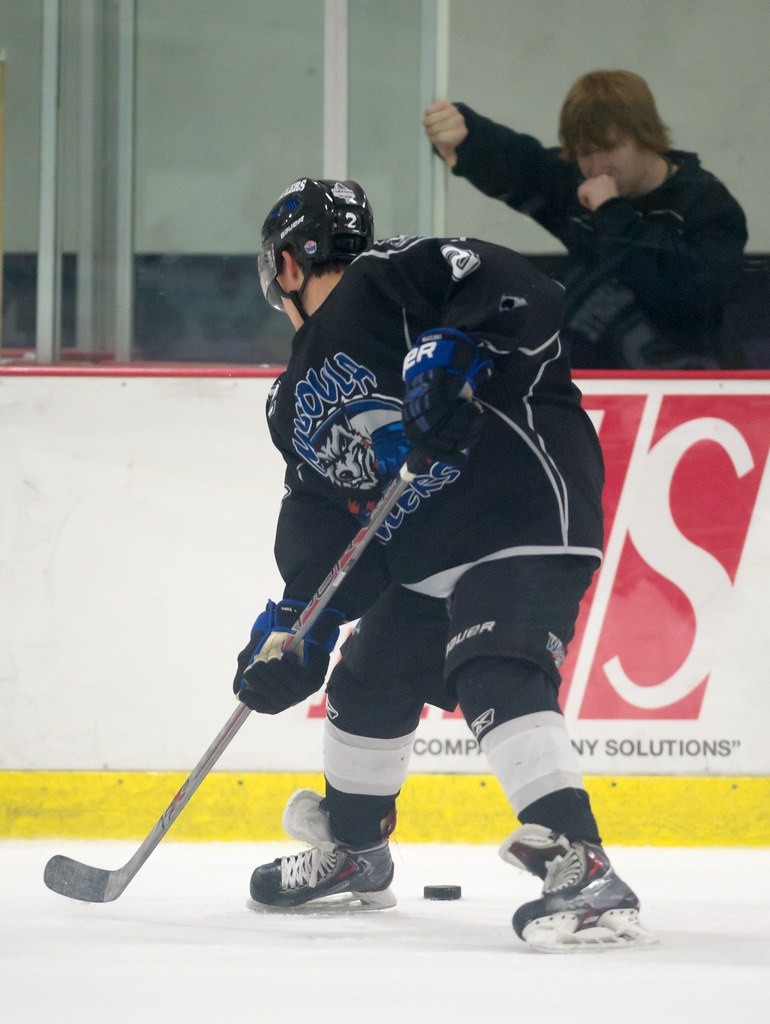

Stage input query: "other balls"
[{"left": 423, "top": 885, "right": 462, "bottom": 901}]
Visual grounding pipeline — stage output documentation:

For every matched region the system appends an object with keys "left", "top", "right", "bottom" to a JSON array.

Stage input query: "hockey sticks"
[{"left": 40, "top": 382, "right": 472, "bottom": 904}]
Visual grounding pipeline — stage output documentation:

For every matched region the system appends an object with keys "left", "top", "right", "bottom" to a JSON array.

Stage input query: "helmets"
[{"left": 257, "top": 177, "right": 375, "bottom": 312}]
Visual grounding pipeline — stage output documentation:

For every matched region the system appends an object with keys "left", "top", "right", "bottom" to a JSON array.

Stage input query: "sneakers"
[
  {"left": 246, "top": 791, "right": 396, "bottom": 915},
  {"left": 499, "top": 823, "right": 660, "bottom": 951}
]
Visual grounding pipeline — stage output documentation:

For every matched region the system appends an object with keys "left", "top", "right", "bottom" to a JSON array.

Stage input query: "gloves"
[
  {"left": 233, "top": 598, "right": 346, "bottom": 715},
  {"left": 403, "top": 327, "right": 498, "bottom": 463}
]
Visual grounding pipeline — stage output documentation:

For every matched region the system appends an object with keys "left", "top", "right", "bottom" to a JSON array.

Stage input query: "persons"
[
  {"left": 422, "top": 70, "right": 748, "bottom": 370},
  {"left": 229, "top": 176, "right": 647, "bottom": 952}
]
